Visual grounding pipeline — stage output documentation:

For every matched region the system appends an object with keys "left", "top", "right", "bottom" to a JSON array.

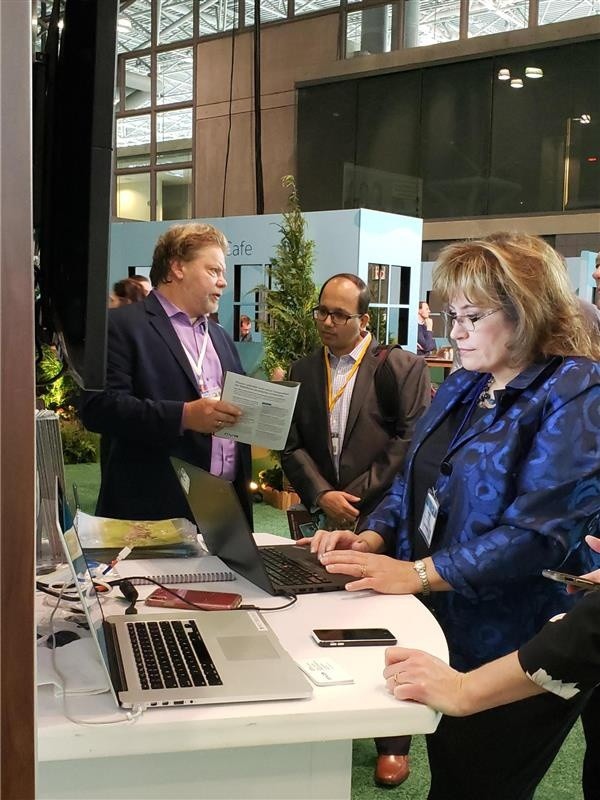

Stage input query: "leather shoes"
[{"left": 375, "top": 755, "right": 408, "bottom": 786}]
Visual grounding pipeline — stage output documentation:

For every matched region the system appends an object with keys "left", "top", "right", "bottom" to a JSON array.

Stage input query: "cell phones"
[
  {"left": 145, "top": 587, "right": 243, "bottom": 611},
  {"left": 312, "top": 628, "right": 397, "bottom": 647},
  {"left": 540, "top": 569, "right": 596, "bottom": 589}
]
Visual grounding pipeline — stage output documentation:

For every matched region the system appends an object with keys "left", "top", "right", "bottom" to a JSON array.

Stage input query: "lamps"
[
  {"left": 497, "top": 67, "right": 544, "bottom": 88},
  {"left": 568, "top": 112, "right": 590, "bottom": 124}
]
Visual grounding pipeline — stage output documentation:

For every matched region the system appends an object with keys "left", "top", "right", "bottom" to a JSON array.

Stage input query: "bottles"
[{"left": 443, "top": 347, "right": 453, "bottom": 360}]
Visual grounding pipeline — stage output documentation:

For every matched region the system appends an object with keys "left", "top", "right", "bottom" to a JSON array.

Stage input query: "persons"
[
  {"left": 292, "top": 231, "right": 600, "bottom": 800},
  {"left": 109, "top": 275, "right": 150, "bottom": 309},
  {"left": 274, "top": 272, "right": 430, "bottom": 787},
  {"left": 241, "top": 315, "right": 254, "bottom": 343},
  {"left": 78, "top": 221, "right": 255, "bottom": 535}
]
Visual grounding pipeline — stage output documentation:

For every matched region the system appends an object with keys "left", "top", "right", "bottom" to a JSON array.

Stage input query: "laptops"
[
  {"left": 168, "top": 456, "right": 365, "bottom": 597},
  {"left": 55, "top": 476, "right": 313, "bottom": 710}
]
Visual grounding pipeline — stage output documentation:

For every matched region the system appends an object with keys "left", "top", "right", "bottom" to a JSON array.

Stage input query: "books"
[
  {"left": 212, "top": 370, "right": 301, "bottom": 451},
  {"left": 111, "top": 555, "right": 236, "bottom": 586}
]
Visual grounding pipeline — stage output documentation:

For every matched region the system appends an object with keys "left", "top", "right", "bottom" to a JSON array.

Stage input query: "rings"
[
  {"left": 341, "top": 518, "right": 345, "bottom": 524},
  {"left": 392, "top": 672, "right": 400, "bottom": 685},
  {"left": 360, "top": 564, "right": 367, "bottom": 578},
  {"left": 215, "top": 421, "right": 221, "bottom": 428}
]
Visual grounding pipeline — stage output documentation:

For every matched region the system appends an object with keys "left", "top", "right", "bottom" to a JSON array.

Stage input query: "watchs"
[{"left": 413, "top": 560, "right": 431, "bottom": 597}]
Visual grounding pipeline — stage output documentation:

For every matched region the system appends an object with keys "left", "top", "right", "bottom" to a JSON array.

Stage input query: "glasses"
[
  {"left": 313, "top": 306, "right": 360, "bottom": 325},
  {"left": 439, "top": 307, "right": 503, "bottom": 332}
]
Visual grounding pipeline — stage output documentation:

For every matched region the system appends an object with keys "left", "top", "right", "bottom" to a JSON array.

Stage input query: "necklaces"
[{"left": 474, "top": 377, "right": 497, "bottom": 410}]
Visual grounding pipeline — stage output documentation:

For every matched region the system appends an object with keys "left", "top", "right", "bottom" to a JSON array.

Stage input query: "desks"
[
  {"left": 33, "top": 531, "right": 454, "bottom": 800},
  {"left": 425, "top": 353, "right": 453, "bottom": 382}
]
